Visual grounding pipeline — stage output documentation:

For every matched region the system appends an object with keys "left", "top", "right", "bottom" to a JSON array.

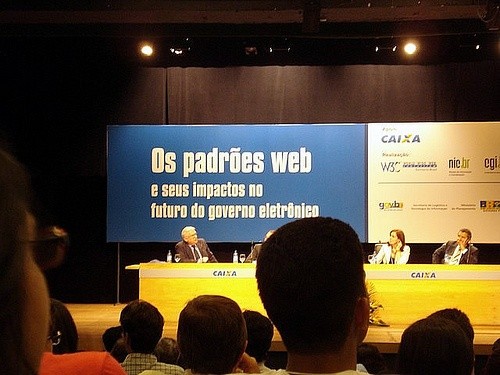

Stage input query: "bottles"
[
  {"left": 233, "top": 250, "right": 238, "bottom": 263},
  {"left": 166, "top": 250, "right": 172, "bottom": 263}
]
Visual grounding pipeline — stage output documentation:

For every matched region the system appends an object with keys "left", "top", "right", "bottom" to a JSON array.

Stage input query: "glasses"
[
  {"left": 46, "top": 331, "right": 61, "bottom": 345},
  {"left": 0, "top": 228, "right": 68, "bottom": 266}
]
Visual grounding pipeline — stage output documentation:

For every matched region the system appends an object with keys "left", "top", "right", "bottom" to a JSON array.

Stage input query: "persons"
[
  {"left": 51, "top": 296, "right": 274, "bottom": 375},
  {"left": 0, "top": 155, "right": 50, "bottom": 374},
  {"left": 358, "top": 309, "right": 500, "bottom": 375},
  {"left": 432, "top": 229, "right": 478, "bottom": 264},
  {"left": 246, "top": 229, "right": 277, "bottom": 260},
  {"left": 367, "top": 229, "right": 411, "bottom": 265},
  {"left": 257, "top": 216, "right": 371, "bottom": 375},
  {"left": 173, "top": 225, "right": 217, "bottom": 263}
]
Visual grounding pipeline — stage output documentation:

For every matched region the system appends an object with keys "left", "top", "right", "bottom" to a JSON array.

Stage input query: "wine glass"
[
  {"left": 240, "top": 254, "right": 245, "bottom": 263},
  {"left": 367, "top": 255, "right": 374, "bottom": 264},
  {"left": 175, "top": 254, "right": 180, "bottom": 263}
]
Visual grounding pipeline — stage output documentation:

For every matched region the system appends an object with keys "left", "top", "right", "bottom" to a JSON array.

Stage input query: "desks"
[{"left": 125, "top": 262, "right": 500, "bottom": 326}]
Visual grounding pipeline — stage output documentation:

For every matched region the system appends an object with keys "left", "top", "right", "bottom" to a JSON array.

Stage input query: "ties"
[{"left": 191, "top": 244, "right": 200, "bottom": 259}]
